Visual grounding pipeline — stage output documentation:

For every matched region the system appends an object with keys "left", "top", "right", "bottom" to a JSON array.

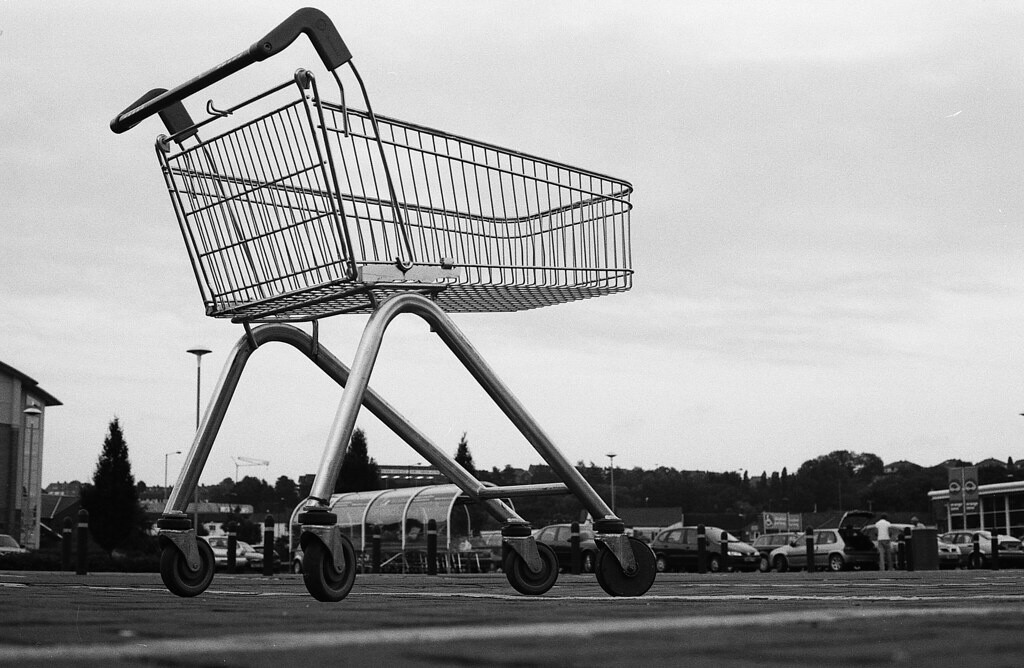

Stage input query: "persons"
[
  {"left": 875, "top": 512, "right": 892, "bottom": 571},
  {"left": 911, "top": 516, "right": 926, "bottom": 530}
]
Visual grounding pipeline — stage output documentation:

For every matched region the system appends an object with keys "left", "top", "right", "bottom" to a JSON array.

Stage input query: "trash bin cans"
[{"left": 910, "top": 527, "right": 938, "bottom": 570}]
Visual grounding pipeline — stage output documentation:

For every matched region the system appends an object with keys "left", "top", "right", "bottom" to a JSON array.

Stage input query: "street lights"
[
  {"left": 186, "top": 350, "right": 212, "bottom": 537},
  {"left": 23, "top": 407, "right": 42, "bottom": 546},
  {"left": 165, "top": 451, "right": 181, "bottom": 507},
  {"left": 606, "top": 455, "right": 616, "bottom": 512}
]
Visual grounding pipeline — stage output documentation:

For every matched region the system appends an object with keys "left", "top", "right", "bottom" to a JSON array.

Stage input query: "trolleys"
[{"left": 109, "top": 7, "right": 658, "bottom": 603}]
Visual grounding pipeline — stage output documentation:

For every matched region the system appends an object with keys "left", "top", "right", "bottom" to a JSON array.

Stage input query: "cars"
[
  {"left": 292, "top": 544, "right": 305, "bottom": 575},
  {"left": 458, "top": 523, "right": 599, "bottom": 574},
  {"left": 941, "top": 531, "right": 1024, "bottom": 570},
  {"left": 250, "top": 545, "right": 281, "bottom": 573},
  {"left": 199, "top": 536, "right": 249, "bottom": 573},
  {"left": 854, "top": 524, "right": 961, "bottom": 570},
  {"left": 238, "top": 542, "right": 265, "bottom": 573},
  {"left": 0, "top": 535, "right": 26, "bottom": 556},
  {"left": 752, "top": 532, "right": 832, "bottom": 573},
  {"left": 650, "top": 526, "right": 761, "bottom": 573},
  {"left": 768, "top": 509, "right": 880, "bottom": 572}
]
